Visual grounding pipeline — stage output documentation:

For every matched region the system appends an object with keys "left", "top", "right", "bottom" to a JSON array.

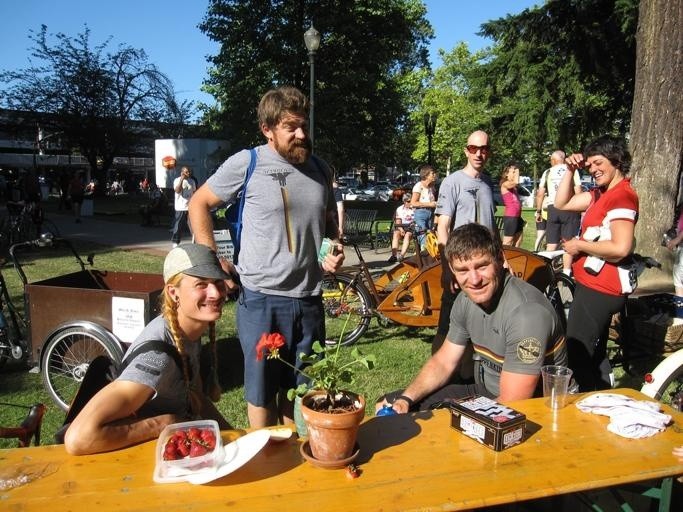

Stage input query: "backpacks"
[
  {"left": 54, "top": 342, "right": 184, "bottom": 443},
  {"left": 224, "top": 201, "right": 245, "bottom": 266}
]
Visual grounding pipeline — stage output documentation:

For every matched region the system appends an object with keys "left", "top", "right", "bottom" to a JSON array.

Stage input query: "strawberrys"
[
  {"left": 344, "top": 465, "right": 359, "bottom": 478},
  {"left": 163, "top": 428, "right": 216, "bottom": 462}
]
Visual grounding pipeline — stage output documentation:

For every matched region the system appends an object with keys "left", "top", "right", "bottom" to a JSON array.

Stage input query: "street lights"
[
  {"left": 302, "top": 20, "right": 321, "bottom": 154},
  {"left": 424, "top": 111, "right": 437, "bottom": 165}
]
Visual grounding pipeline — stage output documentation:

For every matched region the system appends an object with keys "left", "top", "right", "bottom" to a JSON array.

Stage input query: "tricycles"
[{"left": 0, "top": 205, "right": 165, "bottom": 415}]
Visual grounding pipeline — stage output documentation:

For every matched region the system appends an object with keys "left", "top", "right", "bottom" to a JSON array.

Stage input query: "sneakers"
[{"left": 385, "top": 255, "right": 397, "bottom": 266}]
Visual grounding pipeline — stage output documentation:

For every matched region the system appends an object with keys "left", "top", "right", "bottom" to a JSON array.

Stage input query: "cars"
[
  {"left": 492, "top": 178, "right": 597, "bottom": 208},
  {"left": 339, "top": 173, "right": 420, "bottom": 201}
]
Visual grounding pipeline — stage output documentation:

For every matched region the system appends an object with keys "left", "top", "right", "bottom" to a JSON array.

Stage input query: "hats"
[{"left": 160, "top": 240, "right": 233, "bottom": 286}]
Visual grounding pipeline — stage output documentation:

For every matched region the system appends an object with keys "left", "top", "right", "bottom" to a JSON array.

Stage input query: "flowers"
[{"left": 254, "top": 306, "right": 378, "bottom": 407}]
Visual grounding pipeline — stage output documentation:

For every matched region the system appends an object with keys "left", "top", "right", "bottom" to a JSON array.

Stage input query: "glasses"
[{"left": 465, "top": 143, "right": 491, "bottom": 155}]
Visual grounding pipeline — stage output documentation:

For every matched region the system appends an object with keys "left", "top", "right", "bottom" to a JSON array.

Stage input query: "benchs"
[
  {"left": 342, "top": 207, "right": 377, "bottom": 251},
  {"left": 146, "top": 188, "right": 175, "bottom": 227}
]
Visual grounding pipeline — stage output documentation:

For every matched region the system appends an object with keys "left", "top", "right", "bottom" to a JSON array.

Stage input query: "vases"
[{"left": 300, "top": 393, "right": 367, "bottom": 470}]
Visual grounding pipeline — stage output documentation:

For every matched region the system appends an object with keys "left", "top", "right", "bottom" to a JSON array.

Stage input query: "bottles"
[{"left": 663, "top": 233, "right": 678, "bottom": 256}]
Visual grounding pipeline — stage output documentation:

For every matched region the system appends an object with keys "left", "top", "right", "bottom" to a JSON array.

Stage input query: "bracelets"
[
  {"left": 396, "top": 396, "right": 413, "bottom": 409},
  {"left": 537, "top": 210, "right": 542, "bottom": 213}
]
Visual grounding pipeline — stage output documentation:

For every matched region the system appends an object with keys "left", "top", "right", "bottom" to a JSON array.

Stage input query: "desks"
[{"left": 1, "top": 387, "right": 683, "bottom": 512}]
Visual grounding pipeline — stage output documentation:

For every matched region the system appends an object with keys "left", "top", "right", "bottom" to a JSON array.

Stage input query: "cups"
[{"left": 540, "top": 364, "right": 573, "bottom": 410}]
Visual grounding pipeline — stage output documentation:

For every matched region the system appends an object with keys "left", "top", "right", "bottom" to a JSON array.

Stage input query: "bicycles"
[
  {"left": 638, "top": 341, "right": 682, "bottom": 414},
  {"left": 320, "top": 223, "right": 576, "bottom": 350}
]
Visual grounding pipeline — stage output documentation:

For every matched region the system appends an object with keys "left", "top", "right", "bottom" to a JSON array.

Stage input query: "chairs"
[{"left": 1, "top": 401, "right": 46, "bottom": 454}]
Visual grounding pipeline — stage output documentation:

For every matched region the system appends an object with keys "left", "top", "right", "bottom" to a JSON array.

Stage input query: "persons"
[
  {"left": 54, "top": 243, "right": 234, "bottom": 456},
  {"left": 499, "top": 164, "right": 530, "bottom": 247},
  {"left": 553, "top": 133, "right": 637, "bottom": 392},
  {"left": 672, "top": 445, "right": 683, "bottom": 462},
  {"left": 665, "top": 203, "right": 683, "bottom": 300},
  {"left": 536, "top": 150, "right": 585, "bottom": 277},
  {"left": 172, "top": 165, "right": 195, "bottom": 247},
  {"left": 0, "top": 167, "right": 167, "bottom": 228},
  {"left": 431, "top": 131, "right": 516, "bottom": 358},
  {"left": 330, "top": 167, "right": 345, "bottom": 239},
  {"left": 377, "top": 222, "right": 568, "bottom": 416},
  {"left": 189, "top": 85, "right": 346, "bottom": 428},
  {"left": 388, "top": 192, "right": 416, "bottom": 264},
  {"left": 533, "top": 193, "right": 549, "bottom": 251},
  {"left": 410, "top": 165, "right": 437, "bottom": 252}
]
